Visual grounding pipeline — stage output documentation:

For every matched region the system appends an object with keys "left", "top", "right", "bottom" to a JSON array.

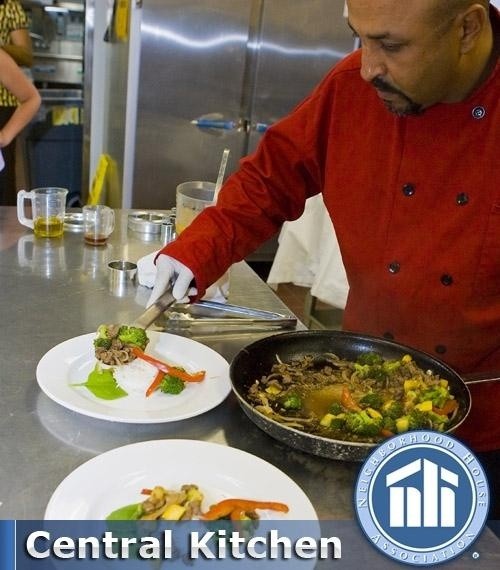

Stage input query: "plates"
[
  {"left": 42, "top": 438, "right": 321, "bottom": 570},
  {"left": 36, "top": 330, "right": 231, "bottom": 424}
]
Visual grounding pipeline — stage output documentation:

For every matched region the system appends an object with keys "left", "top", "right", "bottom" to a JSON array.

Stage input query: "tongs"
[{"left": 155, "top": 299, "right": 298, "bottom": 330}]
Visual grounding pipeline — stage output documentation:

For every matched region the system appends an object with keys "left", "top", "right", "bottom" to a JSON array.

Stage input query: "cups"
[
  {"left": 82, "top": 203, "right": 115, "bottom": 246},
  {"left": 175, "top": 181, "right": 224, "bottom": 238},
  {"left": 16, "top": 186, "right": 69, "bottom": 237}
]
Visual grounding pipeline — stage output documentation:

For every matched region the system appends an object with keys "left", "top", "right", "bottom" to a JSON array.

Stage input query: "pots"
[{"left": 228, "top": 329, "right": 500, "bottom": 465}]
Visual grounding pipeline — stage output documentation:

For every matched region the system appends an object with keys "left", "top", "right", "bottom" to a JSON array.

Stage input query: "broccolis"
[
  {"left": 160, "top": 366, "right": 185, "bottom": 395},
  {"left": 93, "top": 322, "right": 149, "bottom": 352},
  {"left": 282, "top": 351, "right": 447, "bottom": 436}
]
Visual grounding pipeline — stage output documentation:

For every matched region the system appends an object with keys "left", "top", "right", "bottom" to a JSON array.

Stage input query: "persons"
[
  {"left": 141, "top": 0, "right": 497, "bottom": 541},
  {"left": 1, "top": 48, "right": 46, "bottom": 148},
  {"left": 1, "top": 0, "right": 35, "bottom": 209}
]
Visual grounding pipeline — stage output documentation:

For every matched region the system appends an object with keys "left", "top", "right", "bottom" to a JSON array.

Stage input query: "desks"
[{"left": 0, "top": 205, "right": 500, "bottom": 570}]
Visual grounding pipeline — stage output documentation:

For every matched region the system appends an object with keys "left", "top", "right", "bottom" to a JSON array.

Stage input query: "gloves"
[{"left": 145, "top": 253, "right": 198, "bottom": 309}]
